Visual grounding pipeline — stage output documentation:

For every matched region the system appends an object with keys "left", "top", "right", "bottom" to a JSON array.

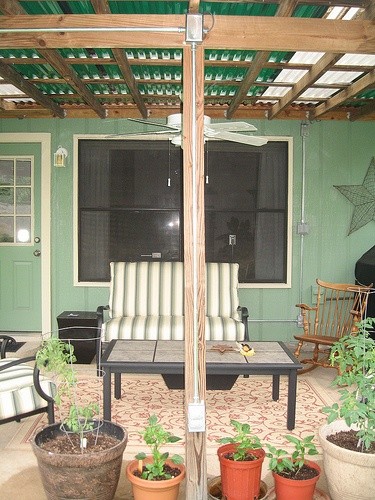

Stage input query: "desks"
[{"left": 97, "top": 339, "right": 302, "bottom": 429}]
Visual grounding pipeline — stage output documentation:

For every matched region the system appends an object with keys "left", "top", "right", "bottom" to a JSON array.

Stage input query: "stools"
[{"left": 57, "top": 312, "right": 100, "bottom": 364}]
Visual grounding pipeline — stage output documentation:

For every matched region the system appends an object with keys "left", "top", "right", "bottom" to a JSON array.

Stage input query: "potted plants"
[
  {"left": 207, "top": 420, "right": 272, "bottom": 500},
  {"left": 321, "top": 319, "right": 375, "bottom": 500},
  {"left": 126, "top": 416, "right": 186, "bottom": 500},
  {"left": 266, "top": 433, "right": 321, "bottom": 500},
  {"left": 30, "top": 339, "right": 128, "bottom": 500}
]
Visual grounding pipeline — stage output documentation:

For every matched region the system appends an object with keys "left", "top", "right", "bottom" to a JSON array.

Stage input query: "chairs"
[
  {"left": 293, "top": 277, "right": 373, "bottom": 386},
  {"left": 0, "top": 335, "right": 54, "bottom": 428}
]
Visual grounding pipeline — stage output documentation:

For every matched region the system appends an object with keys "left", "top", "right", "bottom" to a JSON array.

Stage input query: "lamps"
[
  {"left": 54, "top": 145, "right": 68, "bottom": 167},
  {"left": 171, "top": 134, "right": 182, "bottom": 146}
]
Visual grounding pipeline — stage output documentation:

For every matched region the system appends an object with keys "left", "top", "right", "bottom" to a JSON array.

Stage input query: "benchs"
[{"left": 96, "top": 261, "right": 252, "bottom": 378}]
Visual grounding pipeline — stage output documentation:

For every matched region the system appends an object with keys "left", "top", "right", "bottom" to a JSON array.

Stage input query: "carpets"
[{"left": 6, "top": 372, "right": 340, "bottom": 457}]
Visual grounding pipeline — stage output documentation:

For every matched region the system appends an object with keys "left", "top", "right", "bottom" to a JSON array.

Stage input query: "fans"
[{"left": 113, "top": 113, "right": 268, "bottom": 147}]
